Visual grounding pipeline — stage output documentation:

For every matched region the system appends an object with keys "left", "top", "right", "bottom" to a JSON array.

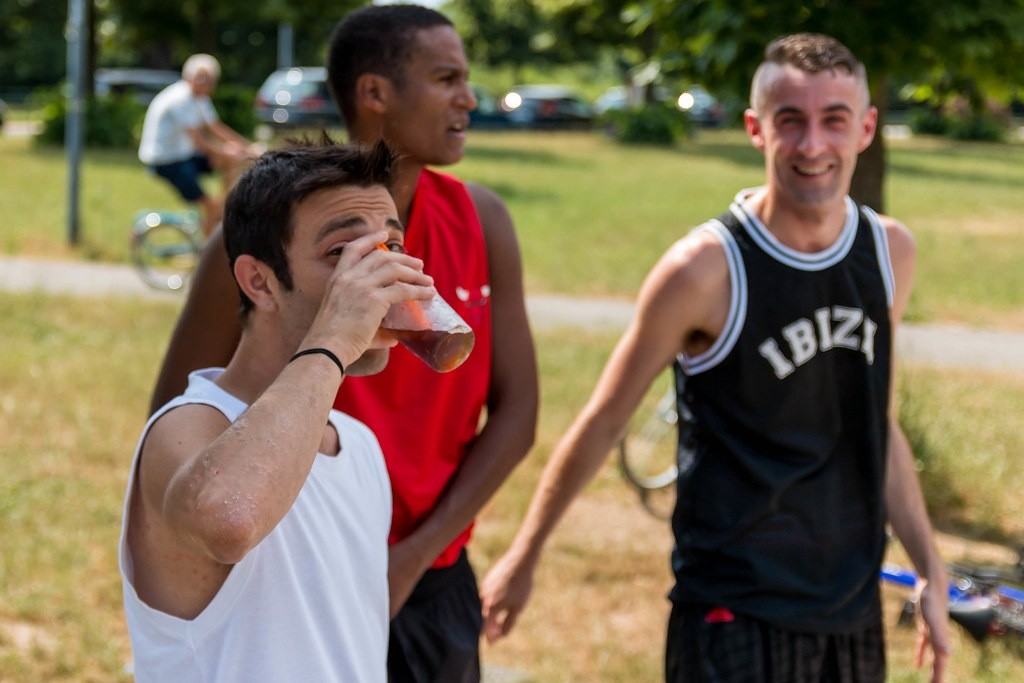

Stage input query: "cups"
[{"left": 380, "top": 271, "right": 475, "bottom": 374}]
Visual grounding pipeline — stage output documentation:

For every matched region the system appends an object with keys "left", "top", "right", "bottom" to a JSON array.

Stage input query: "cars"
[{"left": 95, "top": 66, "right": 725, "bottom": 132}]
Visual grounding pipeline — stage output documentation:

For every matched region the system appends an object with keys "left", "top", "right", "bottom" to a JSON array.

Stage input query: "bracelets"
[{"left": 288, "top": 346, "right": 345, "bottom": 378}]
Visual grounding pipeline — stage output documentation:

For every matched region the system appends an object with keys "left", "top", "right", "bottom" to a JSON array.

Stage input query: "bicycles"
[
  {"left": 619, "top": 363, "right": 678, "bottom": 493},
  {"left": 126, "top": 143, "right": 261, "bottom": 293},
  {"left": 880, "top": 551, "right": 1023, "bottom": 656}
]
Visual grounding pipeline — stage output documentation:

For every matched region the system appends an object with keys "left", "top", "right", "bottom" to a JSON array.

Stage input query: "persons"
[
  {"left": 117, "top": 128, "right": 436, "bottom": 683},
  {"left": 137, "top": 52, "right": 259, "bottom": 235},
  {"left": 147, "top": 5, "right": 540, "bottom": 683},
  {"left": 476, "top": 33, "right": 949, "bottom": 683}
]
